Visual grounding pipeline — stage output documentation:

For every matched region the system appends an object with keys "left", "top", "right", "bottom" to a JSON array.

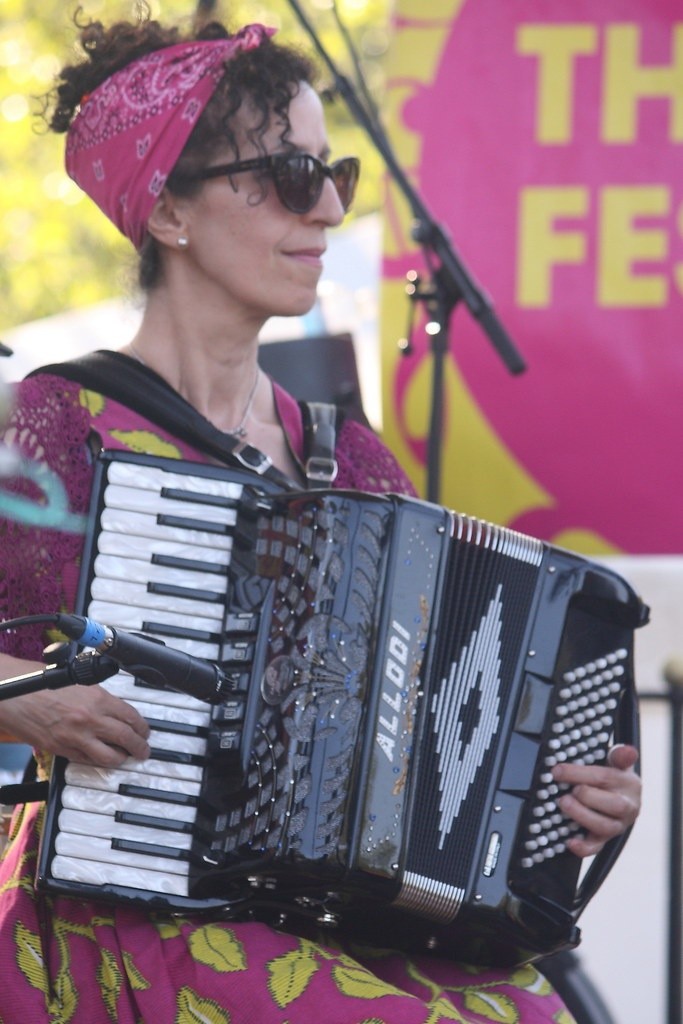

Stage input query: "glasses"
[{"left": 168, "top": 150, "right": 361, "bottom": 217}]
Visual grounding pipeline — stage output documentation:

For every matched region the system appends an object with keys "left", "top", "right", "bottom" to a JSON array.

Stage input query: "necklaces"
[{"left": 223, "top": 366, "right": 261, "bottom": 438}]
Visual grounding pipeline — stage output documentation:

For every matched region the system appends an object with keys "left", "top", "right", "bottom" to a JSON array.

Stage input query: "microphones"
[{"left": 52, "top": 613, "right": 235, "bottom": 707}]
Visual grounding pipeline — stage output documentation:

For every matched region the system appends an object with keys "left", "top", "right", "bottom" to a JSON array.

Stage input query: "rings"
[{"left": 607, "top": 743, "right": 632, "bottom": 771}]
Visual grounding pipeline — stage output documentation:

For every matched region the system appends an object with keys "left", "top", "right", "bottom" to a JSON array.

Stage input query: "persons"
[{"left": 0, "top": 17, "right": 644, "bottom": 1024}]
